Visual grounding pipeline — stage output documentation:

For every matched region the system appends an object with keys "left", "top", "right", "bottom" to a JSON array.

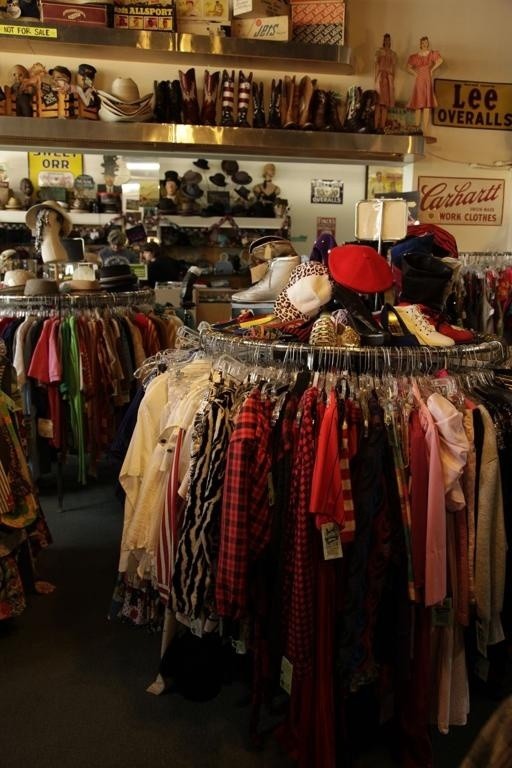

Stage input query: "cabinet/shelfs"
[{"left": 0, "top": 18, "right": 424, "bottom": 230}]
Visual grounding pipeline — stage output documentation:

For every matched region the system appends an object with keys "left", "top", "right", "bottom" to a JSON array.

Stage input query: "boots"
[{"left": 155, "top": 68, "right": 379, "bottom": 132}]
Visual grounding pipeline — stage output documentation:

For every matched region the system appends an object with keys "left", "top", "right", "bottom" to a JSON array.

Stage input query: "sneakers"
[{"left": 211, "top": 231, "right": 474, "bottom": 347}]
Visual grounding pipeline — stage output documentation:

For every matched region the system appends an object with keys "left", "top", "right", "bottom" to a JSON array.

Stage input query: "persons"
[
  {"left": 158, "top": 170, "right": 182, "bottom": 204},
  {"left": 96, "top": 229, "right": 138, "bottom": 266},
  {"left": 404, "top": 36, "right": 444, "bottom": 138},
  {"left": 97, "top": 168, "right": 122, "bottom": 194},
  {"left": 0, "top": 62, "right": 99, "bottom": 120},
  {"left": 252, "top": 164, "right": 280, "bottom": 204},
  {"left": 145, "top": 19, "right": 157, "bottom": 29},
  {"left": 142, "top": 241, "right": 168, "bottom": 288},
  {"left": 373, "top": 32, "right": 398, "bottom": 133},
  {"left": 116, "top": 18, "right": 128, "bottom": 27},
  {"left": 160, "top": 19, "right": 171, "bottom": 28},
  {"left": 129, "top": 20, "right": 142, "bottom": 29},
  {"left": 272, "top": 197, "right": 288, "bottom": 219},
  {"left": 182, "top": 1, "right": 200, "bottom": 16}
]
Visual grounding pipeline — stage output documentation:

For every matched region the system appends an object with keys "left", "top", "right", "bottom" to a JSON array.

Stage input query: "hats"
[
  {"left": 158, "top": 158, "right": 289, "bottom": 217},
  {"left": 0, "top": 199, "right": 139, "bottom": 298},
  {"left": 97, "top": 77, "right": 157, "bottom": 123}
]
